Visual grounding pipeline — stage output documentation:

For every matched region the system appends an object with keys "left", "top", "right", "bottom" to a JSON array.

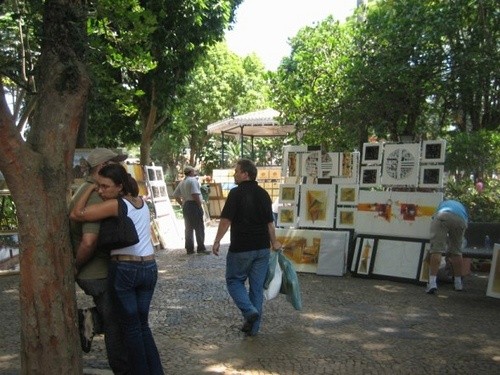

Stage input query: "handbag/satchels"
[
  {"left": 263, "top": 249, "right": 302, "bottom": 312},
  {"left": 99, "top": 196, "right": 140, "bottom": 250}
]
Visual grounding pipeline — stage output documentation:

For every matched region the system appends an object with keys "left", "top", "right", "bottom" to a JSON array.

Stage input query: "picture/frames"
[
  {"left": 72, "top": 148, "right": 122, "bottom": 188},
  {"left": 271, "top": 138, "right": 450, "bottom": 285},
  {"left": 486, "top": 243, "right": 500, "bottom": 298},
  {"left": 144, "top": 165, "right": 169, "bottom": 247}
]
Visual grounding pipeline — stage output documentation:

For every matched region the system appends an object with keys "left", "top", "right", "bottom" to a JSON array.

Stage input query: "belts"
[{"left": 110, "top": 255, "right": 156, "bottom": 261}]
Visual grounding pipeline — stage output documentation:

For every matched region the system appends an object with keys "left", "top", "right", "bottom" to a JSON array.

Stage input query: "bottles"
[{"left": 484, "top": 235, "right": 490, "bottom": 251}]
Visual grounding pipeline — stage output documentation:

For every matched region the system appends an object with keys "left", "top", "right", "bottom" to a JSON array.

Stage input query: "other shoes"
[
  {"left": 241, "top": 312, "right": 260, "bottom": 333},
  {"left": 197, "top": 250, "right": 211, "bottom": 254},
  {"left": 77, "top": 308, "right": 96, "bottom": 354},
  {"left": 425, "top": 281, "right": 439, "bottom": 293},
  {"left": 187, "top": 250, "right": 195, "bottom": 254},
  {"left": 455, "top": 283, "right": 464, "bottom": 290}
]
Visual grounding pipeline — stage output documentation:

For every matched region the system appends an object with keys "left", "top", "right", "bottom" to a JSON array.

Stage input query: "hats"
[
  {"left": 184, "top": 166, "right": 195, "bottom": 174},
  {"left": 87, "top": 147, "right": 128, "bottom": 168}
]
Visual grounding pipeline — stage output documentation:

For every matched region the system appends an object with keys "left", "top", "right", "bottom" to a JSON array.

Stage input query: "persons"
[
  {"left": 71, "top": 164, "right": 166, "bottom": 375},
  {"left": 68, "top": 147, "right": 127, "bottom": 353},
  {"left": 213, "top": 158, "right": 281, "bottom": 336},
  {"left": 173, "top": 166, "right": 212, "bottom": 255},
  {"left": 426, "top": 200, "right": 468, "bottom": 293}
]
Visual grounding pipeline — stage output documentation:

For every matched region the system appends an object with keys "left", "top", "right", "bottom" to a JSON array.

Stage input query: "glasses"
[{"left": 94, "top": 183, "right": 117, "bottom": 191}]
